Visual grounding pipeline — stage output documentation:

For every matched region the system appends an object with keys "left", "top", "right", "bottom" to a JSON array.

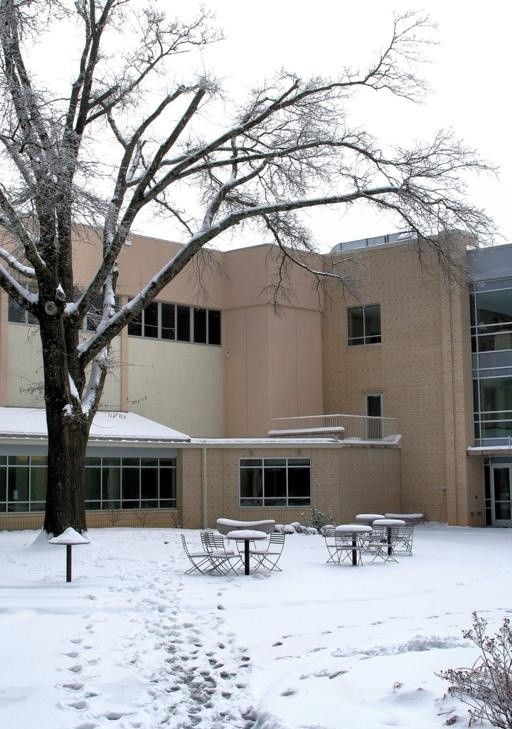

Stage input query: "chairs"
[
  {"left": 324, "top": 518, "right": 416, "bottom": 566},
  {"left": 181, "top": 531, "right": 286, "bottom": 576}
]
